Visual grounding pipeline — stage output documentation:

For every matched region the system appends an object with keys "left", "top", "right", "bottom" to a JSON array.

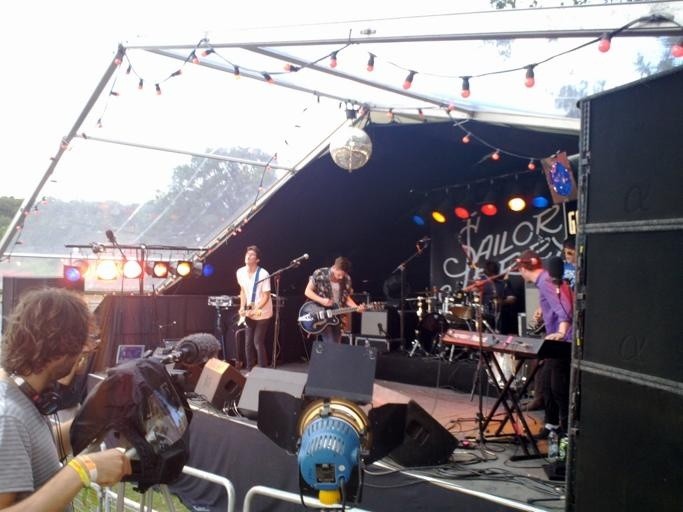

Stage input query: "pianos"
[{"left": 444, "top": 328, "right": 571, "bottom": 360}]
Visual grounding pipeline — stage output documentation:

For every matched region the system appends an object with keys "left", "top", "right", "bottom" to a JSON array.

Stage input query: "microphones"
[
  {"left": 419, "top": 235, "right": 433, "bottom": 243},
  {"left": 292, "top": 252, "right": 310, "bottom": 265},
  {"left": 512, "top": 255, "right": 541, "bottom": 268},
  {"left": 377, "top": 321, "right": 383, "bottom": 332}
]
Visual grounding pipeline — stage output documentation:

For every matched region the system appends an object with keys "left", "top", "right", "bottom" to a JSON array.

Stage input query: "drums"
[{"left": 419, "top": 313, "right": 471, "bottom": 359}]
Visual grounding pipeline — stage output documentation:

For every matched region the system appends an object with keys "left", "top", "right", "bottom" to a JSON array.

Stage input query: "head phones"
[{"left": 9, "top": 369, "right": 63, "bottom": 416}]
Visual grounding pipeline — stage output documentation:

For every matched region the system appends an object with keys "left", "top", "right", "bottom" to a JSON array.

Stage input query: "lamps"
[
  {"left": 199, "top": 252, "right": 216, "bottom": 279},
  {"left": 121, "top": 254, "right": 143, "bottom": 280},
  {"left": 453, "top": 184, "right": 477, "bottom": 219},
  {"left": 95, "top": 260, "right": 118, "bottom": 281},
  {"left": 431, "top": 187, "right": 456, "bottom": 225},
  {"left": 175, "top": 253, "right": 193, "bottom": 279},
  {"left": 503, "top": 173, "right": 527, "bottom": 215},
  {"left": 63, "top": 246, "right": 89, "bottom": 282},
  {"left": 477, "top": 179, "right": 501, "bottom": 217},
  {"left": 531, "top": 169, "right": 551, "bottom": 211},
  {"left": 255, "top": 341, "right": 409, "bottom": 512},
  {"left": 328, "top": 104, "right": 372, "bottom": 173},
  {"left": 412, "top": 191, "right": 434, "bottom": 226},
  {"left": 151, "top": 253, "right": 169, "bottom": 278}
]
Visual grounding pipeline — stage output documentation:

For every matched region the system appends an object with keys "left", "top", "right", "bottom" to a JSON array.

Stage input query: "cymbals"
[
  {"left": 414, "top": 289, "right": 444, "bottom": 294},
  {"left": 449, "top": 303, "right": 474, "bottom": 320}
]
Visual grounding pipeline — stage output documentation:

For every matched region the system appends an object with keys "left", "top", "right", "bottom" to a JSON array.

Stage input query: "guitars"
[
  {"left": 299, "top": 302, "right": 387, "bottom": 335},
  {"left": 237, "top": 303, "right": 263, "bottom": 328}
]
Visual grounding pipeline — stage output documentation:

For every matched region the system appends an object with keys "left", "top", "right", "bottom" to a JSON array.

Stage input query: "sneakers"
[
  {"left": 522, "top": 399, "right": 543, "bottom": 411},
  {"left": 455, "top": 352, "right": 470, "bottom": 360},
  {"left": 532, "top": 423, "right": 565, "bottom": 440}
]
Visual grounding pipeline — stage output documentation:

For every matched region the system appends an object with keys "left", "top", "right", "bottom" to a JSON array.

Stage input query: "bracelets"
[
  {"left": 76, "top": 454, "right": 100, "bottom": 482},
  {"left": 66, "top": 459, "right": 90, "bottom": 487}
]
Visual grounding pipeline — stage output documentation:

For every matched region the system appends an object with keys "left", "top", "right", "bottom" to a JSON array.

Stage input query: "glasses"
[{"left": 562, "top": 252, "right": 574, "bottom": 255}]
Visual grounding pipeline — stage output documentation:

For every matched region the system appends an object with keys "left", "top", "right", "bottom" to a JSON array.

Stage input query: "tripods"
[{"left": 460, "top": 307, "right": 520, "bottom": 451}]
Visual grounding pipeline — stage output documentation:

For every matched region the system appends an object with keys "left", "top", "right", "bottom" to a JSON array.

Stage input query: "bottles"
[{"left": 548, "top": 427, "right": 559, "bottom": 463}]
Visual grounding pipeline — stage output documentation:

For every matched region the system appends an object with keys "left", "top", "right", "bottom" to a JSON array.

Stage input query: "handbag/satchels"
[{"left": 419, "top": 312, "right": 447, "bottom": 335}]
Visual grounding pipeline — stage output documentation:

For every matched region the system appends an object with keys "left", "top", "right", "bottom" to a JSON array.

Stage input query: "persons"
[
  {"left": 477, "top": 259, "right": 518, "bottom": 335},
  {"left": 235, "top": 244, "right": 273, "bottom": 378},
  {"left": 514, "top": 249, "right": 573, "bottom": 441},
  {"left": 532, "top": 235, "right": 576, "bottom": 323},
  {"left": 303, "top": 256, "right": 367, "bottom": 344},
  {"left": 0, "top": 284, "right": 133, "bottom": 512}
]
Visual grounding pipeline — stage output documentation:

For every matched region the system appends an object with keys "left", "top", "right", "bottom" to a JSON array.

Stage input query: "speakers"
[
  {"left": 390, "top": 399, "right": 459, "bottom": 468},
  {"left": 302, "top": 340, "right": 379, "bottom": 404},
  {"left": 237, "top": 365, "right": 309, "bottom": 419},
  {"left": 193, "top": 357, "right": 245, "bottom": 407}
]
